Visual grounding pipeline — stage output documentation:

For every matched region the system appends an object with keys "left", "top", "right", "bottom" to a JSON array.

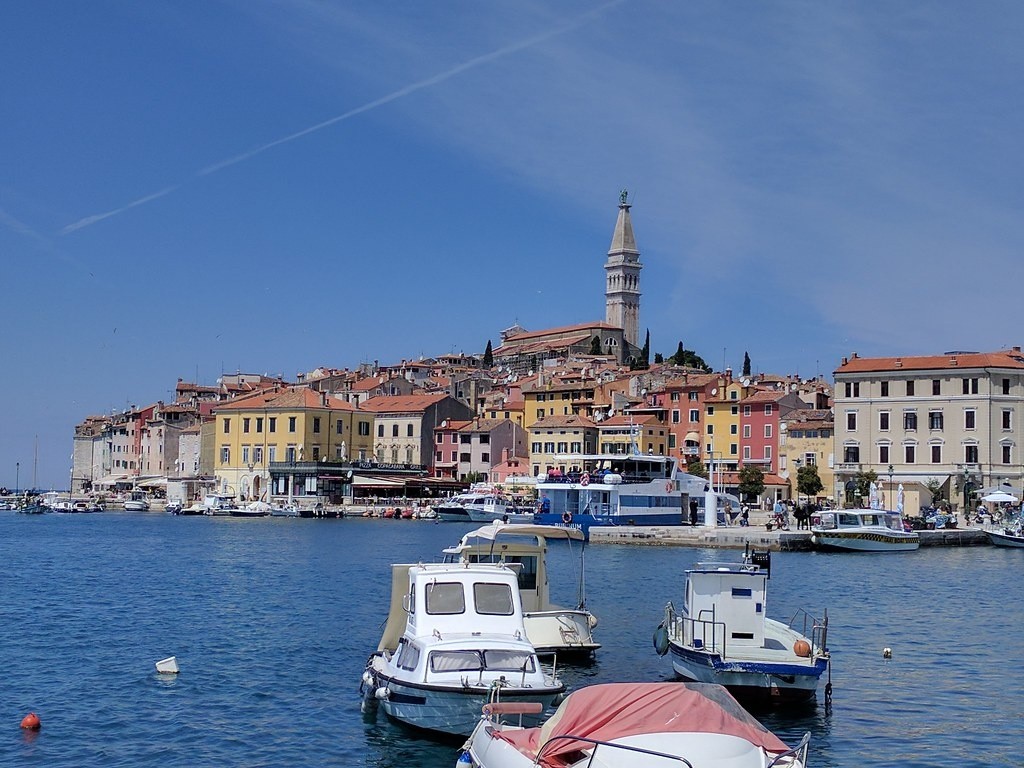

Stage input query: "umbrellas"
[
  {"left": 870, "top": 480, "right": 904, "bottom": 512},
  {"left": 981, "top": 491, "right": 1018, "bottom": 509},
  {"left": 969, "top": 485, "right": 1023, "bottom": 494}
]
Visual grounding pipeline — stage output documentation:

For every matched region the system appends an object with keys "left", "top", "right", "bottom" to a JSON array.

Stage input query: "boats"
[
  {"left": 270, "top": 497, "right": 302, "bottom": 517},
  {"left": 361, "top": 540, "right": 567, "bottom": 742},
  {"left": 74, "top": 502, "right": 107, "bottom": 513},
  {"left": 43, "top": 489, "right": 58, "bottom": 508},
  {"left": 0, "top": 496, "right": 25, "bottom": 509},
  {"left": 297, "top": 501, "right": 345, "bottom": 518},
  {"left": 179, "top": 493, "right": 240, "bottom": 515},
  {"left": 973, "top": 517, "right": 1024, "bottom": 549},
  {"left": 652, "top": 541, "right": 833, "bottom": 699},
  {"left": 229, "top": 500, "right": 273, "bottom": 517},
  {"left": 165, "top": 495, "right": 183, "bottom": 512},
  {"left": 124, "top": 487, "right": 151, "bottom": 511},
  {"left": 428, "top": 467, "right": 509, "bottom": 522},
  {"left": 440, "top": 518, "right": 602, "bottom": 655},
  {"left": 363, "top": 493, "right": 438, "bottom": 519},
  {"left": 505, "top": 492, "right": 538, "bottom": 517},
  {"left": 808, "top": 509, "right": 920, "bottom": 550},
  {"left": 51, "top": 496, "right": 74, "bottom": 511},
  {"left": 532, "top": 416, "right": 742, "bottom": 541},
  {"left": 456, "top": 681, "right": 812, "bottom": 767}
]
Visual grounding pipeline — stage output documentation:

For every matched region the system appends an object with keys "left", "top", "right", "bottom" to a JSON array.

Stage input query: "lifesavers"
[
  {"left": 503, "top": 515, "right": 508, "bottom": 522},
  {"left": 580, "top": 474, "right": 590, "bottom": 485},
  {"left": 664, "top": 483, "right": 673, "bottom": 493},
  {"left": 562, "top": 512, "right": 572, "bottom": 523}
]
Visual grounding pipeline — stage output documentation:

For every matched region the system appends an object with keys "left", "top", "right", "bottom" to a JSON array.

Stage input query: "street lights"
[
  {"left": 15, "top": 434, "right": 51, "bottom": 513},
  {"left": 70, "top": 467, "right": 74, "bottom": 502},
  {"left": 795, "top": 457, "right": 804, "bottom": 507},
  {"left": 964, "top": 469, "right": 970, "bottom": 514},
  {"left": 888, "top": 464, "right": 896, "bottom": 511},
  {"left": 16, "top": 462, "right": 19, "bottom": 496}
]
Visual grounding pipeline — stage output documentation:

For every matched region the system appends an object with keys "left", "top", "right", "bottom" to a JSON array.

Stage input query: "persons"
[
  {"left": 149, "top": 487, "right": 165, "bottom": 499},
  {"left": 766, "top": 495, "right": 834, "bottom": 530},
  {"left": 193, "top": 490, "right": 199, "bottom": 501},
  {"left": 373, "top": 494, "right": 378, "bottom": 504},
  {"left": 741, "top": 503, "right": 750, "bottom": 527},
  {"left": 689, "top": 498, "right": 698, "bottom": 528},
  {"left": 510, "top": 556, "right": 525, "bottom": 589},
  {"left": 402, "top": 494, "right": 407, "bottom": 505},
  {"left": 724, "top": 503, "right": 733, "bottom": 528},
  {"left": 95, "top": 495, "right": 105, "bottom": 507},
  {"left": 293, "top": 500, "right": 298, "bottom": 512},
  {"left": 541, "top": 495, "right": 551, "bottom": 514},
  {"left": 549, "top": 467, "right": 650, "bottom": 485},
  {"left": 921, "top": 499, "right": 1024, "bottom": 536},
  {"left": 26, "top": 496, "right": 31, "bottom": 507},
  {"left": 229, "top": 499, "right": 238, "bottom": 509}
]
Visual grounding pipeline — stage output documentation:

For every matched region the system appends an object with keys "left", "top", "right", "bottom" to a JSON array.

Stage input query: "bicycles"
[{"left": 768, "top": 510, "right": 789, "bottom": 531}]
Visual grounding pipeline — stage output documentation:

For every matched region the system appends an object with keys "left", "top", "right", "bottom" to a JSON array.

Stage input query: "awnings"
[{"left": 91, "top": 474, "right": 127, "bottom": 485}]
